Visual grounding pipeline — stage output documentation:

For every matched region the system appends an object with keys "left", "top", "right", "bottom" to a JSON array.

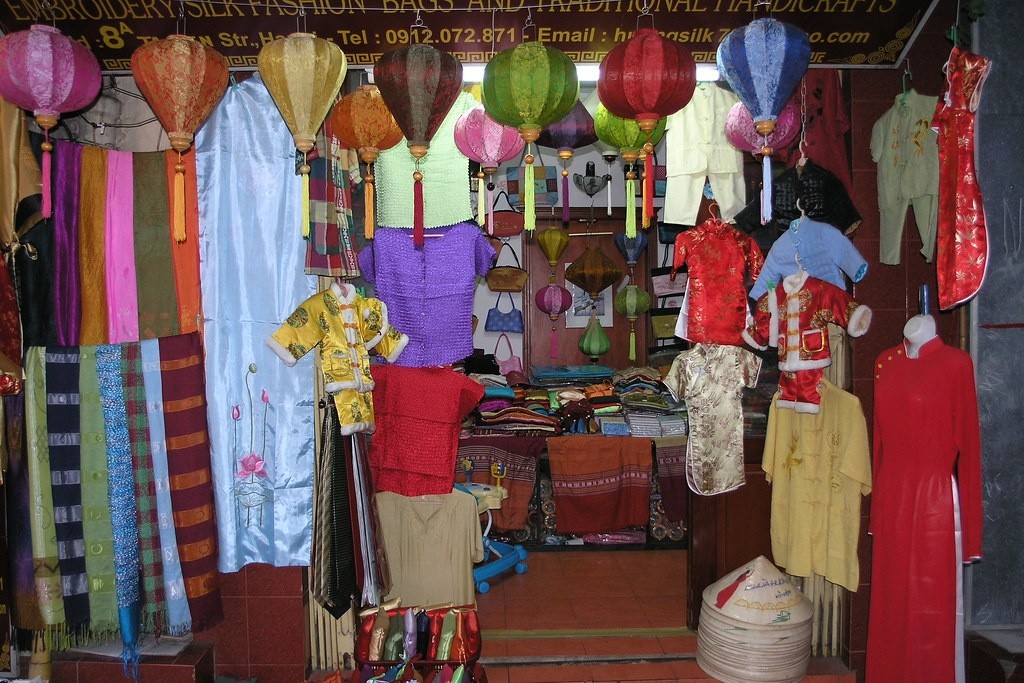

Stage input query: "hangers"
[{"left": 323, "top": 20, "right": 960, "bottom": 296}]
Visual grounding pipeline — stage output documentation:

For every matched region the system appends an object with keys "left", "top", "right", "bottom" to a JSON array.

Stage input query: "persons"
[{"left": 865, "top": 312, "right": 984, "bottom": 683}]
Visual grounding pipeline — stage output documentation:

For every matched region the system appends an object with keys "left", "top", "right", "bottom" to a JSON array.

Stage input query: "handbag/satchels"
[
  {"left": 484, "top": 291, "right": 525, "bottom": 334},
  {"left": 658, "top": 221, "right": 692, "bottom": 245},
  {"left": 506, "top": 141, "right": 558, "bottom": 207},
  {"left": 485, "top": 243, "right": 529, "bottom": 292},
  {"left": 651, "top": 243, "right": 688, "bottom": 298},
  {"left": 492, "top": 334, "right": 523, "bottom": 376},
  {"left": 649, "top": 298, "right": 681, "bottom": 339},
  {"left": 647, "top": 339, "right": 688, "bottom": 367},
  {"left": 483, "top": 191, "right": 524, "bottom": 237},
  {"left": 624, "top": 149, "right": 666, "bottom": 198}
]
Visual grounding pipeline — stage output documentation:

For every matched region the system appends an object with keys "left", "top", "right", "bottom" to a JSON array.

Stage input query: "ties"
[{"left": 309, "top": 395, "right": 391, "bottom": 620}]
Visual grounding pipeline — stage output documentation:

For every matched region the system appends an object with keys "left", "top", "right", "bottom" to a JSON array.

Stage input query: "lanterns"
[
  {"left": 597, "top": 27, "right": 697, "bottom": 220},
  {"left": 482, "top": 37, "right": 580, "bottom": 235},
  {"left": 257, "top": 32, "right": 348, "bottom": 238},
  {"left": 373, "top": 41, "right": 467, "bottom": 248},
  {"left": 129, "top": 34, "right": 229, "bottom": 244},
  {"left": 329, "top": 80, "right": 404, "bottom": 240},
  {"left": 615, "top": 226, "right": 650, "bottom": 285},
  {"left": 725, "top": 95, "right": 806, "bottom": 226},
  {"left": 613, "top": 284, "right": 652, "bottom": 362},
  {"left": 535, "top": 282, "right": 573, "bottom": 358},
  {"left": 537, "top": 224, "right": 569, "bottom": 284},
  {"left": 592, "top": 100, "right": 669, "bottom": 238},
  {"left": 564, "top": 245, "right": 623, "bottom": 331},
  {"left": 714, "top": 17, "right": 812, "bottom": 223},
  {"left": 453, "top": 99, "right": 525, "bottom": 236},
  {"left": 578, "top": 318, "right": 612, "bottom": 367},
  {"left": 0, "top": 22, "right": 104, "bottom": 221},
  {"left": 535, "top": 96, "right": 599, "bottom": 222}
]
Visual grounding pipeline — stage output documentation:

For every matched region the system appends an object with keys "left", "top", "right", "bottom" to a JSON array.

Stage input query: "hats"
[{"left": 696, "top": 555, "right": 815, "bottom": 683}]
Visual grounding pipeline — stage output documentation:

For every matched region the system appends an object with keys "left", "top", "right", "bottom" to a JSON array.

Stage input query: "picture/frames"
[{"left": 563, "top": 262, "right": 614, "bottom": 328}]
